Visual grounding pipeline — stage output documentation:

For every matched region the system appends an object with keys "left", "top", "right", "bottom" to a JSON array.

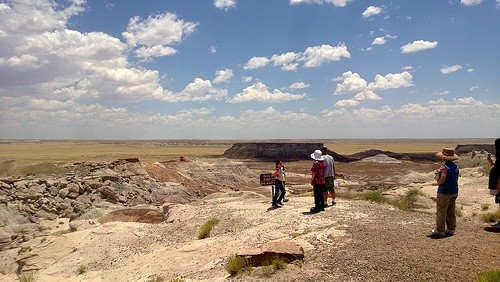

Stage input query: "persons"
[
  {"left": 488, "top": 138, "right": 500, "bottom": 227},
  {"left": 309, "top": 149, "right": 327, "bottom": 214},
  {"left": 320, "top": 146, "right": 337, "bottom": 208},
  {"left": 272, "top": 159, "right": 289, "bottom": 208},
  {"left": 429, "top": 147, "right": 460, "bottom": 237}
]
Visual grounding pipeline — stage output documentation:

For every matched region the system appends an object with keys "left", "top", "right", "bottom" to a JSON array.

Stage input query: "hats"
[
  {"left": 435, "top": 146, "right": 458, "bottom": 161},
  {"left": 310, "top": 150, "right": 324, "bottom": 161}
]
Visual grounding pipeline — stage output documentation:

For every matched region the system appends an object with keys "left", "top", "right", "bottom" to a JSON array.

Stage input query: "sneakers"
[
  {"left": 484, "top": 220, "right": 500, "bottom": 232},
  {"left": 311, "top": 201, "right": 336, "bottom": 212},
  {"left": 272, "top": 199, "right": 289, "bottom": 207},
  {"left": 432, "top": 229, "right": 455, "bottom": 236}
]
